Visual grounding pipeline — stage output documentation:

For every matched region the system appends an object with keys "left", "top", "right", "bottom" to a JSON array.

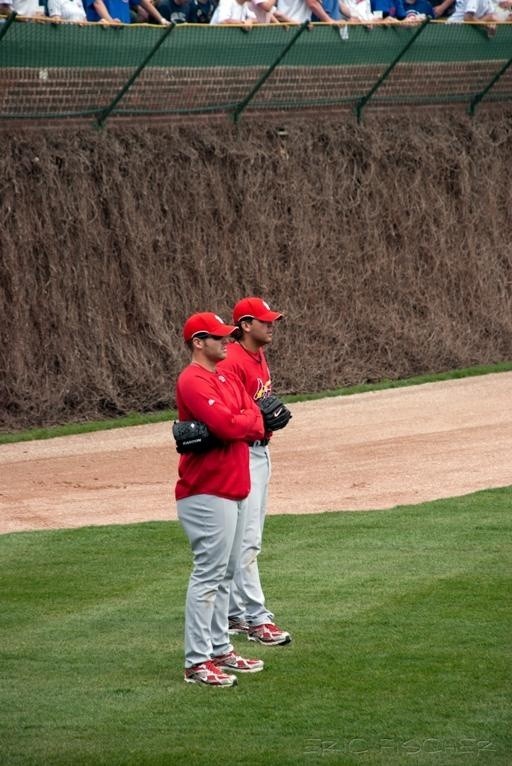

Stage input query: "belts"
[{"left": 248, "top": 438, "right": 269, "bottom": 447}]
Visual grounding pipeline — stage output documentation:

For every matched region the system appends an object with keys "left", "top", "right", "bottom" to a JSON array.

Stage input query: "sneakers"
[
  {"left": 183, "top": 663, "right": 237, "bottom": 686},
  {"left": 229, "top": 621, "right": 291, "bottom": 646},
  {"left": 212, "top": 653, "right": 264, "bottom": 674}
]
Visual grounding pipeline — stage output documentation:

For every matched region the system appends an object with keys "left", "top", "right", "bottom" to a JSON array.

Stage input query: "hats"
[
  {"left": 182, "top": 311, "right": 236, "bottom": 343},
  {"left": 232, "top": 296, "right": 283, "bottom": 324}
]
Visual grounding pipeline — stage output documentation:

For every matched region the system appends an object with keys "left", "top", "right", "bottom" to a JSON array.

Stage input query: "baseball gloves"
[
  {"left": 173, "top": 422, "right": 213, "bottom": 453},
  {"left": 256, "top": 394, "right": 291, "bottom": 432}
]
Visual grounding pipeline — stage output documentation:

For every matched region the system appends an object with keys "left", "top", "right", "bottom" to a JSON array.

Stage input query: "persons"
[
  {"left": 171, "top": 310, "right": 266, "bottom": 691},
  {"left": 0, "top": 1, "right": 511, "bottom": 37},
  {"left": 218, "top": 296, "right": 295, "bottom": 647}
]
